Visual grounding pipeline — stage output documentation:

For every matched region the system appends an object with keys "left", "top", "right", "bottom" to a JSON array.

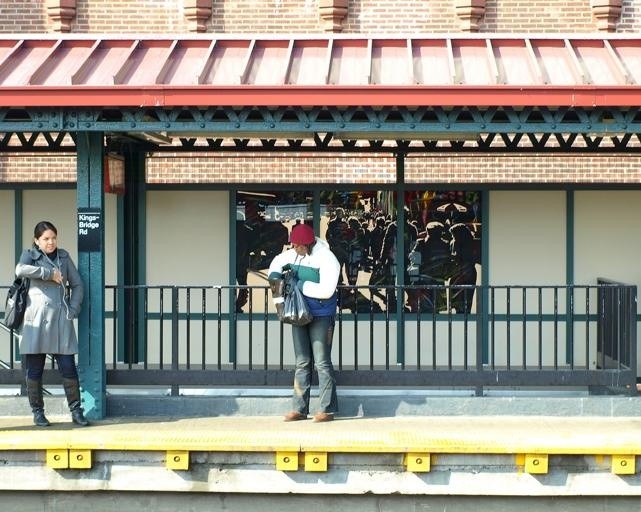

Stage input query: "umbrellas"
[{"left": 437, "top": 203, "right": 468, "bottom": 212}]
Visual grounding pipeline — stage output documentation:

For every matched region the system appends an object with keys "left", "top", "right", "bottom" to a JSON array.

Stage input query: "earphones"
[{"left": 36, "top": 240, "right": 39, "bottom": 245}]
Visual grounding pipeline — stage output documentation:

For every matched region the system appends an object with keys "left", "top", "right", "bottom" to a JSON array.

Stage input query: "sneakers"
[
  {"left": 69, "top": 407, "right": 90, "bottom": 426},
  {"left": 312, "top": 412, "right": 334, "bottom": 422},
  {"left": 283, "top": 410, "right": 306, "bottom": 422},
  {"left": 32, "top": 408, "right": 50, "bottom": 427}
]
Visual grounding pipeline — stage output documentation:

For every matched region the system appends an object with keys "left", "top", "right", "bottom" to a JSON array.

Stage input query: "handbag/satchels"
[{"left": 4, "top": 278, "right": 30, "bottom": 329}]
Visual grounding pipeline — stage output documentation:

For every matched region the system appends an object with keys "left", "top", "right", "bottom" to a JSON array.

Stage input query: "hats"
[{"left": 290, "top": 224, "right": 315, "bottom": 245}]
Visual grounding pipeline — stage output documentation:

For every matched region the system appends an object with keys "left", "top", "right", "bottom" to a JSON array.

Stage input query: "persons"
[
  {"left": 16, "top": 221, "right": 90, "bottom": 427},
  {"left": 268, "top": 224, "right": 342, "bottom": 422},
  {"left": 236, "top": 208, "right": 477, "bottom": 313}
]
